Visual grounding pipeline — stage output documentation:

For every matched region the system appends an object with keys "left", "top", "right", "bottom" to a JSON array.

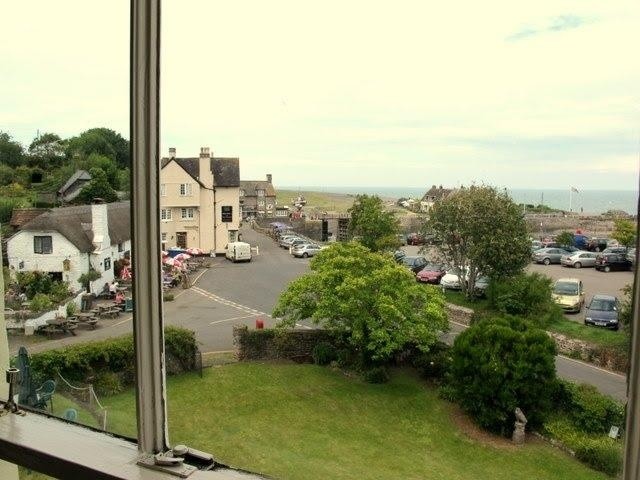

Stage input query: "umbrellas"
[{"left": 120, "top": 246, "right": 204, "bottom": 281}]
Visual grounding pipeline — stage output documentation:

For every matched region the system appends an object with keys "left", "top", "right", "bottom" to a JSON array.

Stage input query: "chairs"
[{"left": 38, "top": 278, "right": 133, "bottom": 340}]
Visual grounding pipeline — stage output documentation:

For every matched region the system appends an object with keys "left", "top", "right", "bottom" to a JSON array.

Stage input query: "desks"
[
  {"left": 162, "top": 281, "right": 172, "bottom": 291},
  {"left": 188, "top": 262, "right": 198, "bottom": 271}
]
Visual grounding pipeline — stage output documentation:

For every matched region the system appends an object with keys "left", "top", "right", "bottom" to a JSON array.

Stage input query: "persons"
[
  {"left": 116, "top": 292, "right": 126, "bottom": 313},
  {"left": 109, "top": 282, "right": 116, "bottom": 300}
]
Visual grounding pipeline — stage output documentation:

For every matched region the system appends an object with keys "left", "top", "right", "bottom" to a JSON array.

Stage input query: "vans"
[{"left": 225, "top": 241, "right": 252, "bottom": 263}]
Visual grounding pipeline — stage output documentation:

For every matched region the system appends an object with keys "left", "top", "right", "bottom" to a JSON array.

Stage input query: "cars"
[
  {"left": 531, "top": 234, "right": 636, "bottom": 271},
  {"left": 551, "top": 278, "right": 623, "bottom": 331},
  {"left": 268, "top": 221, "right": 322, "bottom": 258},
  {"left": 391, "top": 252, "right": 490, "bottom": 297},
  {"left": 398, "top": 233, "right": 435, "bottom": 246}
]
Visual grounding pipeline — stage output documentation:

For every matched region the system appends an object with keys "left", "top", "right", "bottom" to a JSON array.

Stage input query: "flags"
[{"left": 571, "top": 186, "right": 579, "bottom": 194}]
